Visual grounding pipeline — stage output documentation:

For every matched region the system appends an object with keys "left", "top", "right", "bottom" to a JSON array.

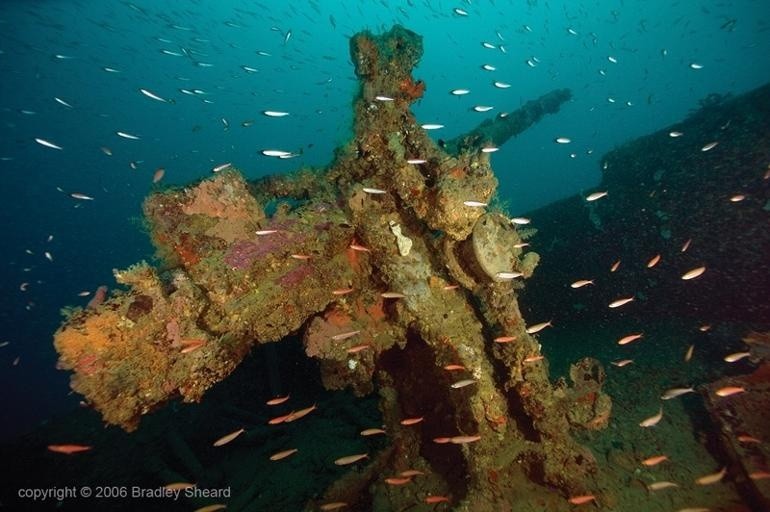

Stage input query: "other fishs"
[{"left": 0, "top": 0, "right": 770, "bottom": 512}]
